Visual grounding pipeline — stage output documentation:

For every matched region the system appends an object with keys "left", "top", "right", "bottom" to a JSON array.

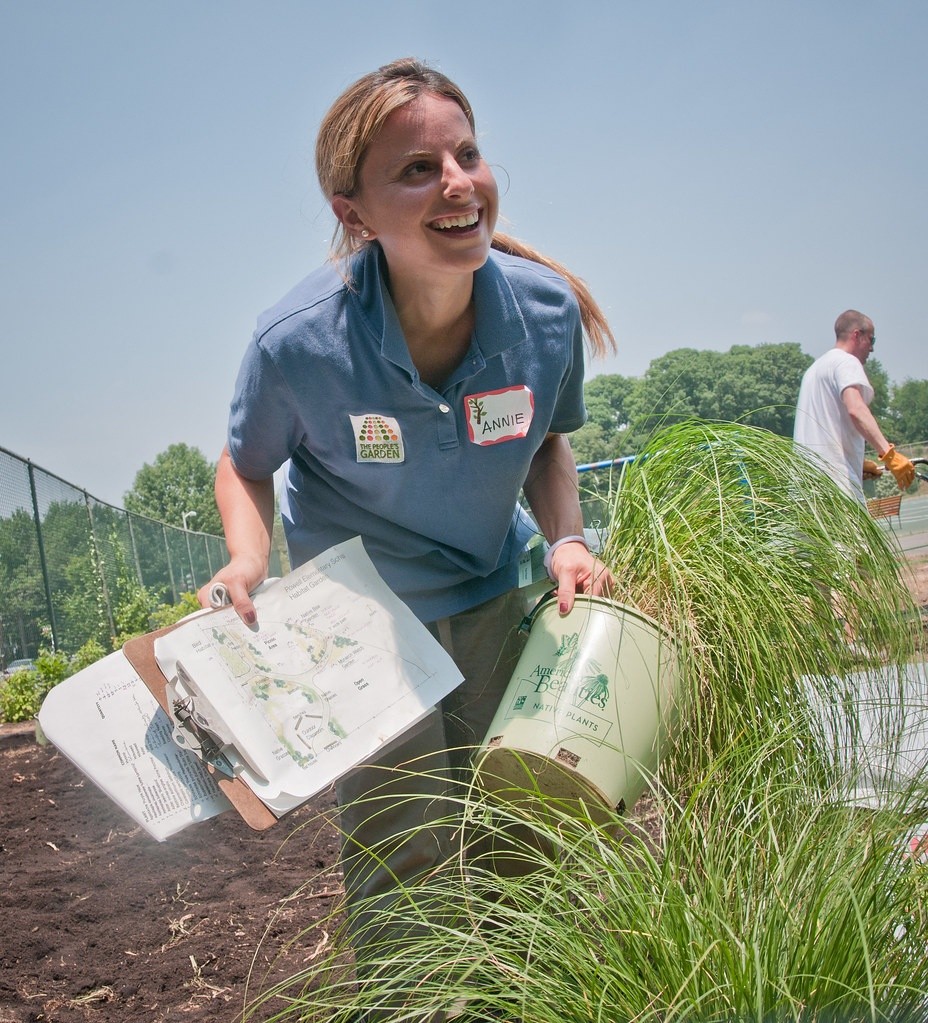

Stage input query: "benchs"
[{"left": 866, "top": 492, "right": 903, "bottom": 531}]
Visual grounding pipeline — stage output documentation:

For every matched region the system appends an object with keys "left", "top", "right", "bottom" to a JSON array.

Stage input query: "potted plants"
[{"left": 472, "top": 418, "right": 925, "bottom": 839}]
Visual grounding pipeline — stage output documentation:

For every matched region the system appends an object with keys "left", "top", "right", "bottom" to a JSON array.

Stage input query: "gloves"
[{"left": 877, "top": 444, "right": 917, "bottom": 489}]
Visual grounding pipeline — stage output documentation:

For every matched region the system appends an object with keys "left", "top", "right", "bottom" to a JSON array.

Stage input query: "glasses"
[{"left": 856, "top": 330, "right": 876, "bottom": 345}]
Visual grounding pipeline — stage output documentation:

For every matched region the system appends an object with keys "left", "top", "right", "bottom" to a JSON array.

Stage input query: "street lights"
[{"left": 182, "top": 511, "right": 197, "bottom": 592}]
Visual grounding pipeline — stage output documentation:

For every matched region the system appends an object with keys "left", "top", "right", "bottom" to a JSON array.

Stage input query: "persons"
[
  {"left": 794, "top": 308, "right": 916, "bottom": 663},
  {"left": 196, "top": 61, "right": 617, "bottom": 1023}
]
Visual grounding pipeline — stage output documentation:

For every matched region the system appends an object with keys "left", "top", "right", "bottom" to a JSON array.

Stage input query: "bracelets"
[{"left": 542, "top": 535, "right": 591, "bottom": 585}]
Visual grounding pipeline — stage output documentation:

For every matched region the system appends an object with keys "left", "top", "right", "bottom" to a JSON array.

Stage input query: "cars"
[{"left": 0, "top": 658, "right": 39, "bottom": 679}]
